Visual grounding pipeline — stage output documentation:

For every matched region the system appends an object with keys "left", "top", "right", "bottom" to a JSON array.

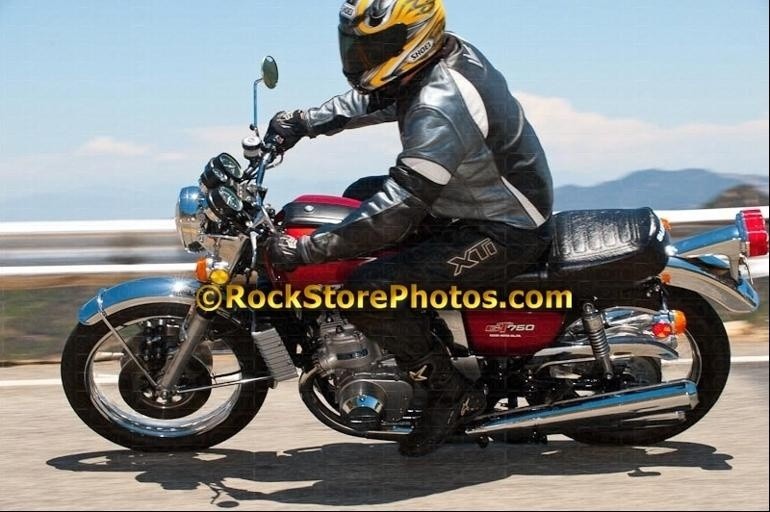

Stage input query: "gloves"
[
  {"left": 263, "top": 109, "right": 306, "bottom": 154},
  {"left": 264, "top": 233, "right": 310, "bottom": 271}
]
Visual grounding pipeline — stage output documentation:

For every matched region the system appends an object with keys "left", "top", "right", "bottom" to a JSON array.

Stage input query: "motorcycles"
[{"left": 59, "top": 56, "right": 768, "bottom": 453}]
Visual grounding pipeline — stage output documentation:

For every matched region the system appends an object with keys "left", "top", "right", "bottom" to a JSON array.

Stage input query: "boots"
[{"left": 397, "top": 335, "right": 488, "bottom": 457}]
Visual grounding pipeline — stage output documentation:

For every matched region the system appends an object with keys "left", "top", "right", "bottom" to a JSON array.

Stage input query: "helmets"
[{"left": 338, "top": 0, "right": 447, "bottom": 95}]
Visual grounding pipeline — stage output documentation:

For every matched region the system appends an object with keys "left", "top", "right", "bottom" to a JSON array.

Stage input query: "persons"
[{"left": 262, "top": 0, "right": 555, "bottom": 457}]
